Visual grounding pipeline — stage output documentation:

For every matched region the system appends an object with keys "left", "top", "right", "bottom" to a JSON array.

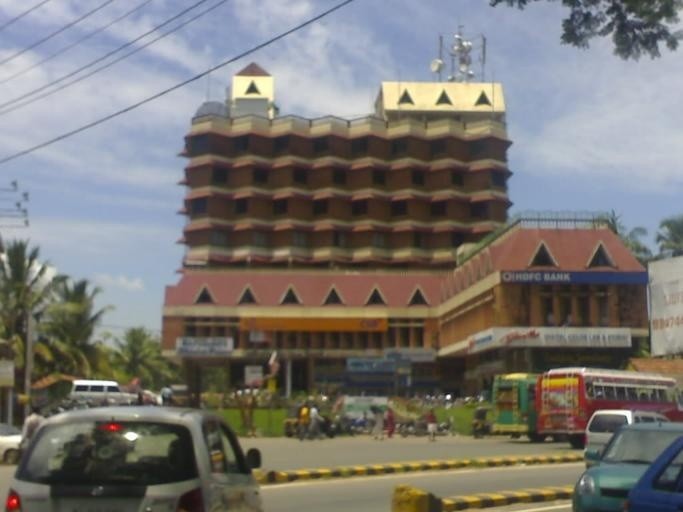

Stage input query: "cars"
[
  {"left": 0, "top": 380, "right": 261, "bottom": 512},
  {"left": 573, "top": 409, "right": 683, "bottom": 511}
]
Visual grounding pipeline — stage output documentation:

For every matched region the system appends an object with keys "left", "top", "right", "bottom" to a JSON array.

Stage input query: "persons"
[
  {"left": 426, "top": 409, "right": 438, "bottom": 441},
  {"left": 159, "top": 385, "right": 172, "bottom": 405},
  {"left": 18, "top": 404, "right": 47, "bottom": 446},
  {"left": 296, "top": 402, "right": 395, "bottom": 440}
]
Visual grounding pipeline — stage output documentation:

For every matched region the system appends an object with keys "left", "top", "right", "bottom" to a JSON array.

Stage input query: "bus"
[{"left": 492, "top": 367, "right": 682, "bottom": 448}]
